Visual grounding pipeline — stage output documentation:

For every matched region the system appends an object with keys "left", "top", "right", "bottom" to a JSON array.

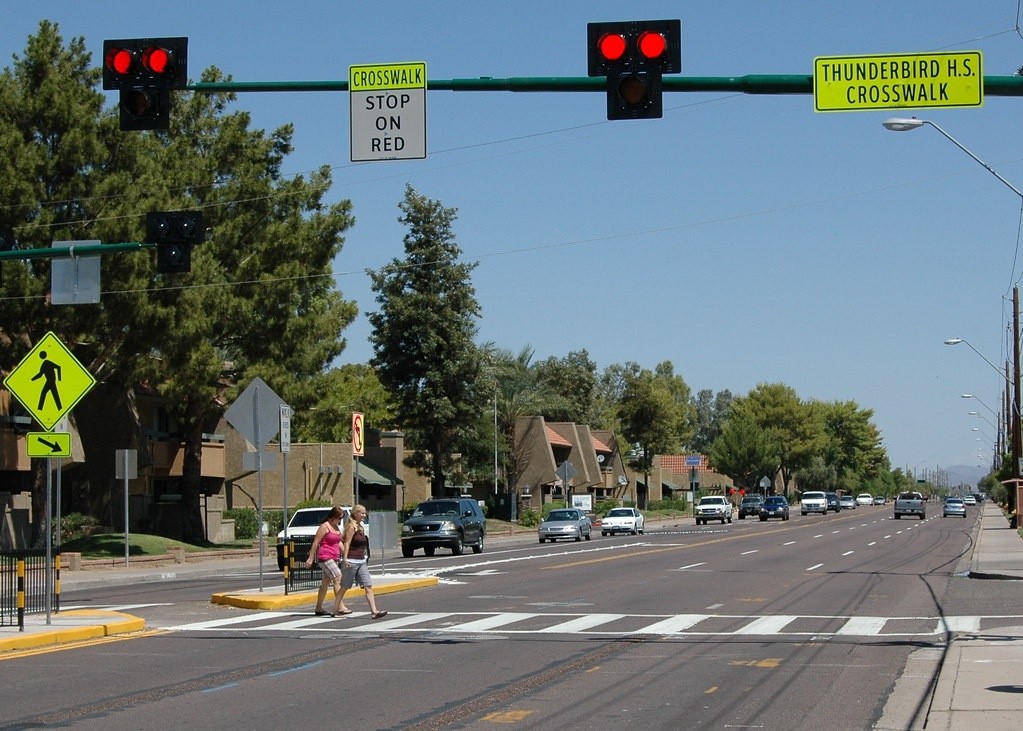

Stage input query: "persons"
[
  {"left": 331, "top": 505, "right": 387, "bottom": 619},
  {"left": 306, "top": 508, "right": 353, "bottom": 614}
]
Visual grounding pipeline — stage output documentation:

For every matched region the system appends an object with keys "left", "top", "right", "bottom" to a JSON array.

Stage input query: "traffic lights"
[
  {"left": 103, "top": 37, "right": 188, "bottom": 131},
  {"left": 588, "top": 19, "right": 681, "bottom": 120}
]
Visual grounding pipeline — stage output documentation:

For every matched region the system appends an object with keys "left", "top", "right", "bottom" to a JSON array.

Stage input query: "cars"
[
  {"left": 943, "top": 498, "right": 966, "bottom": 518},
  {"left": 972, "top": 494, "right": 981, "bottom": 502},
  {"left": 538, "top": 508, "right": 592, "bottom": 543},
  {"left": 964, "top": 496, "right": 976, "bottom": 505},
  {"left": 601, "top": 508, "right": 644, "bottom": 536},
  {"left": 857, "top": 494, "right": 874, "bottom": 505},
  {"left": 840, "top": 495, "right": 858, "bottom": 509},
  {"left": 873, "top": 496, "right": 885, "bottom": 505}
]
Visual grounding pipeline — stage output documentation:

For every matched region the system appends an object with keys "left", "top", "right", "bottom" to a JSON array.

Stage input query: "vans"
[{"left": 801, "top": 491, "right": 828, "bottom": 515}]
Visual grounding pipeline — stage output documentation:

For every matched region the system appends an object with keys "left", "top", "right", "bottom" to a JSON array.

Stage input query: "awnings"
[{"left": 352, "top": 458, "right": 405, "bottom": 486}]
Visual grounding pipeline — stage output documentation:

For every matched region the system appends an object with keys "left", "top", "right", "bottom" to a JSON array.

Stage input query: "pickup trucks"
[
  {"left": 893, "top": 491, "right": 928, "bottom": 519},
  {"left": 694, "top": 496, "right": 732, "bottom": 525}
]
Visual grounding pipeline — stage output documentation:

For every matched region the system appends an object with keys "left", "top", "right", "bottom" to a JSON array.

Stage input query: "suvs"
[
  {"left": 278, "top": 507, "right": 371, "bottom": 571},
  {"left": 401, "top": 497, "right": 487, "bottom": 558},
  {"left": 738, "top": 496, "right": 789, "bottom": 521},
  {"left": 825, "top": 492, "right": 841, "bottom": 513}
]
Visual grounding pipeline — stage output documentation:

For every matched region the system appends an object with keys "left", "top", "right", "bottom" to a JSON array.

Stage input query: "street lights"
[
  {"left": 961, "top": 394, "right": 1015, "bottom": 514},
  {"left": 969, "top": 411, "right": 1006, "bottom": 472},
  {"left": 944, "top": 338, "right": 1022, "bottom": 529}
]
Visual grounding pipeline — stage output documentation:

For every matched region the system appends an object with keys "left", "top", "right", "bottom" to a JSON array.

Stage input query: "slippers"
[
  {"left": 372, "top": 610, "right": 387, "bottom": 619},
  {"left": 330, "top": 611, "right": 344, "bottom": 618},
  {"left": 340, "top": 609, "right": 353, "bottom": 614},
  {"left": 315, "top": 610, "right": 331, "bottom": 615}
]
